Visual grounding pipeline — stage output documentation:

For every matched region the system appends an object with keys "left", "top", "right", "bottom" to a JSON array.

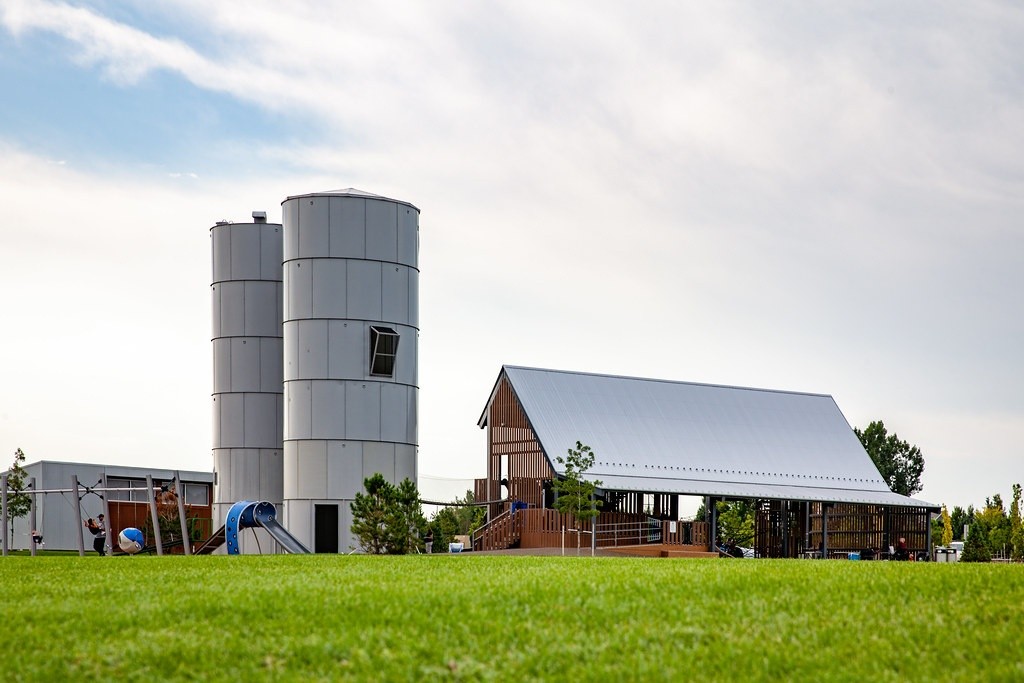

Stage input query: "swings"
[
  {"left": 61, "top": 492, "right": 100, "bottom": 534},
  {"left": 21, "top": 494, "right": 45, "bottom": 544}
]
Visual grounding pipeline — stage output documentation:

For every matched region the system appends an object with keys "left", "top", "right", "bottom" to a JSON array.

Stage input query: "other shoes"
[
  {"left": 101, "top": 531, "right": 104, "bottom": 536},
  {"left": 99, "top": 553, "right": 106, "bottom": 556}
]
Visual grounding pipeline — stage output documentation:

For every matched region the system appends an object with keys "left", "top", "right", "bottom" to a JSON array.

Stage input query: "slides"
[{"left": 256, "top": 517, "right": 311, "bottom": 554}]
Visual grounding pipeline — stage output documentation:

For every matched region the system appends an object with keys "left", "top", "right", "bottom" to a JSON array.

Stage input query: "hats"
[{"left": 97, "top": 514, "right": 104, "bottom": 518}]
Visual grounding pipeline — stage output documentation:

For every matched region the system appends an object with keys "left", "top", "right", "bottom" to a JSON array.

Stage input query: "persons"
[
  {"left": 715, "top": 535, "right": 722, "bottom": 551},
  {"left": 84, "top": 518, "right": 105, "bottom": 535},
  {"left": 32, "top": 531, "right": 45, "bottom": 544},
  {"left": 93, "top": 514, "right": 106, "bottom": 556},
  {"left": 726, "top": 538, "right": 744, "bottom": 558}
]
